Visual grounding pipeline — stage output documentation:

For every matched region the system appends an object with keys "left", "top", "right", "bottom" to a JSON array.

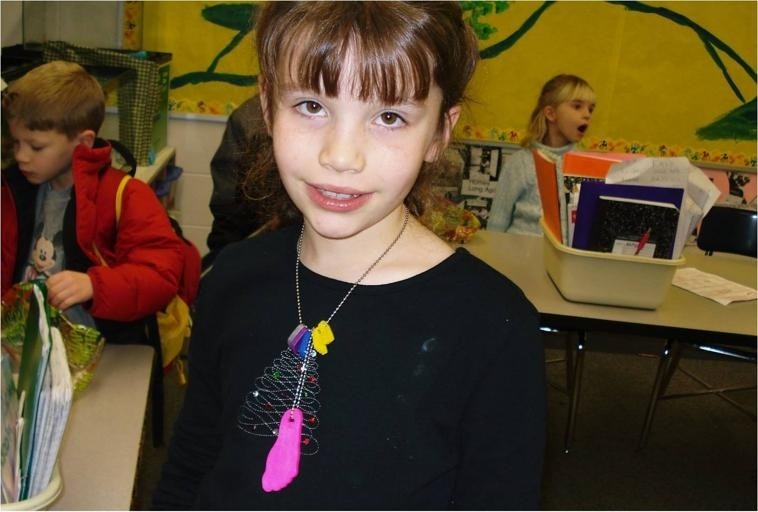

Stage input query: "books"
[
  {"left": 0, "top": 283, "right": 73, "bottom": 504},
  {"left": 530, "top": 148, "right": 721, "bottom": 259}
]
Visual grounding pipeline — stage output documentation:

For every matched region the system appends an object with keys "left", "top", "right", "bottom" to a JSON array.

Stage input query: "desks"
[
  {"left": 50, "top": 345, "right": 157, "bottom": 509},
  {"left": 436, "top": 230, "right": 756, "bottom": 449}
]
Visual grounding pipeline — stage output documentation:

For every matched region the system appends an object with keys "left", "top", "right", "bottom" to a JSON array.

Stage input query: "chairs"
[{"left": 698, "top": 209, "right": 758, "bottom": 258}]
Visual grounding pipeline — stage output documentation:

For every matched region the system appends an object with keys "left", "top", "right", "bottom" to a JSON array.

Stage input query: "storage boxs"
[{"left": 535, "top": 222, "right": 685, "bottom": 315}]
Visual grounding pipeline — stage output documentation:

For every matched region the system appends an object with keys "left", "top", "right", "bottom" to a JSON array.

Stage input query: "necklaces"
[{"left": 237, "top": 207, "right": 409, "bottom": 494}]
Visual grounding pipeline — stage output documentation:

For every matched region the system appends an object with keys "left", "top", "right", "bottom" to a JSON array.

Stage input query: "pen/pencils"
[{"left": 634, "top": 228, "right": 651, "bottom": 256}]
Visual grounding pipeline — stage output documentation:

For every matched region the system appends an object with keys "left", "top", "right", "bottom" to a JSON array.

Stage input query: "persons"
[
  {"left": 487, "top": 74, "right": 596, "bottom": 236},
  {"left": 130, "top": 1, "right": 547, "bottom": 511},
  {"left": 0, "top": 60, "right": 202, "bottom": 341}
]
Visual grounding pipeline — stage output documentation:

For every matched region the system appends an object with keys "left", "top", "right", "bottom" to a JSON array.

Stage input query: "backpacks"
[{"left": 115, "top": 176, "right": 193, "bottom": 368}]
